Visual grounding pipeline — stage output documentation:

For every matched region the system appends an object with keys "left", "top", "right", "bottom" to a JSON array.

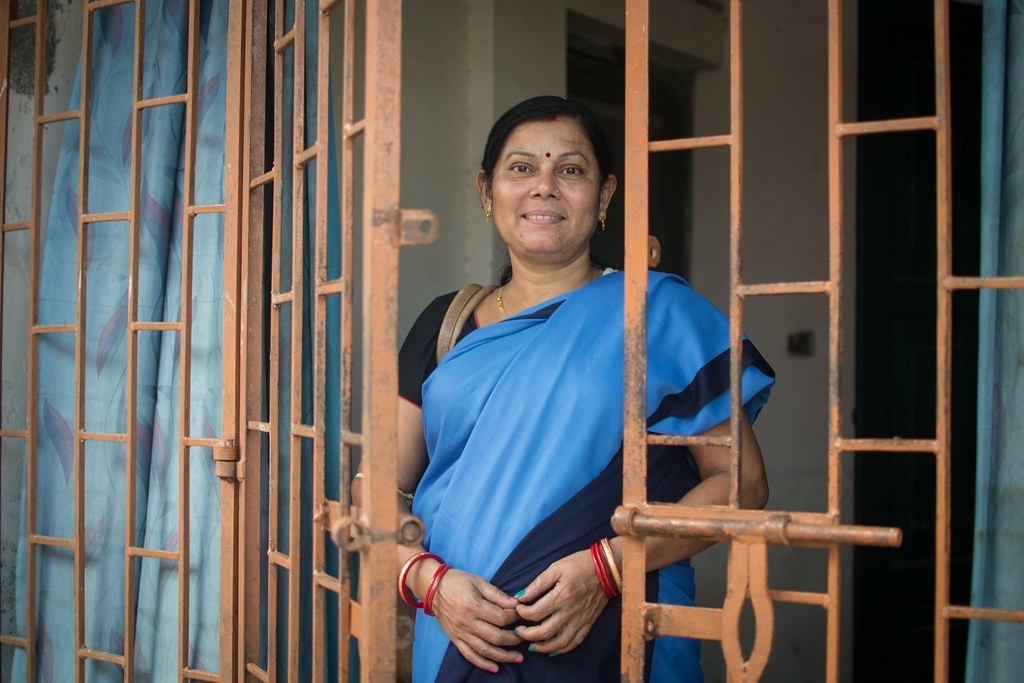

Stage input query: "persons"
[{"left": 350, "top": 95, "right": 776, "bottom": 683}]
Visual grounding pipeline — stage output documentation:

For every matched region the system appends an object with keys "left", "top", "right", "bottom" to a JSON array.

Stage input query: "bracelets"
[
  {"left": 590, "top": 536, "right": 622, "bottom": 601},
  {"left": 397, "top": 552, "right": 452, "bottom": 617}
]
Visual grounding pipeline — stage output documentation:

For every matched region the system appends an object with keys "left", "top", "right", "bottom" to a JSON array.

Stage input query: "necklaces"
[{"left": 497, "top": 268, "right": 604, "bottom": 321}]
